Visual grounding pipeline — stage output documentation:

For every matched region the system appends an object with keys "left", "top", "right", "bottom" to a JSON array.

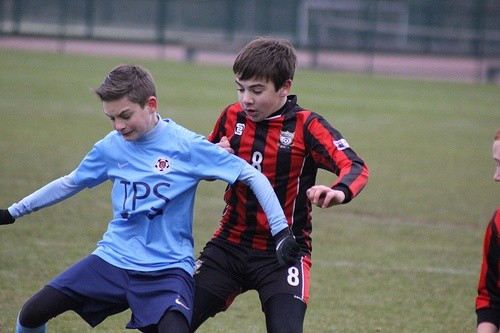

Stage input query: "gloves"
[
  {"left": 0, "top": 210, "right": 16, "bottom": 226},
  {"left": 273, "top": 227, "right": 302, "bottom": 267}
]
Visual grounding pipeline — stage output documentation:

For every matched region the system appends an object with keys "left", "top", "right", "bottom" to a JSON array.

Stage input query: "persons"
[
  {"left": 0, "top": 64, "right": 300, "bottom": 333},
  {"left": 138, "top": 38, "right": 369, "bottom": 333},
  {"left": 475, "top": 129, "right": 500, "bottom": 333}
]
